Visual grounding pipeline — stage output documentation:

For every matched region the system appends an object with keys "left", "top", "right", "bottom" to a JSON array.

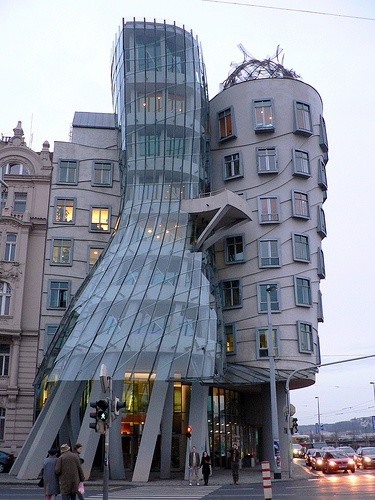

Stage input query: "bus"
[{"left": 292, "top": 434, "right": 312, "bottom": 445}]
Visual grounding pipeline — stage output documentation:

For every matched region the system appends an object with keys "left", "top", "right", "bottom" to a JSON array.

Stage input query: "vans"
[{"left": 301, "top": 442, "right": 328, "bottom": 449}]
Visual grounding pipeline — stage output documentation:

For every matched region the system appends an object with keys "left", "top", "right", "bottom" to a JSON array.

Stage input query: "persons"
[
  {"left": 55, "top": 444, "right": 85, "bottom": 500},
  {"left": 200, "top": 451, "right": 212, "bottom": 485},
  {"left": 42, "top": 448, "right": 61, "bottom": 500},
  {"left": 188, "top": 446, "right": 200, "bottom": 486},
  {"left": 226, "top": 442, "right": 240, "bottom": 485},
  {"left": 328, "top": 454, "right": 333, "bottom": 458},
  {"left": 72, "top": 443, "right": 84, "bottom": 464}
]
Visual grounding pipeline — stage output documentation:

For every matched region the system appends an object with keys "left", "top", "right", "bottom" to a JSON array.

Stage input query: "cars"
[
  {"left": 291, "top": 444, "right": 304, "bottom": 458},
  {"left": 305, "top": 446, "right": 375, "bottom": 474},
  {"left": 0, "top": 451, "right": 15, "bottom": 473}
]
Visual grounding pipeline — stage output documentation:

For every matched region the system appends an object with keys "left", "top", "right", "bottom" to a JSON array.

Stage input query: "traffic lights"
[
  {"left": 89, "top": 398, "right": 108, "bottom": 434},
  {"left": 114, "top": 396, "right": 126, "bottom": 417}
]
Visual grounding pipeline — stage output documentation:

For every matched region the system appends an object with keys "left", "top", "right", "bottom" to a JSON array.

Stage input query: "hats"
[
  {"left": 48, "top": 449, "right": 57, "bottom": 456},
  {"left": 60, "top": 444, "right": 70, "bottom": 452},
  {"left": 75, "top": 444, "right": 82, "bottom": 449}
]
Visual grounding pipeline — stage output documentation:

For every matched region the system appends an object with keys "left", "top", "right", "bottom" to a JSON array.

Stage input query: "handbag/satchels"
[
  {"left": 78, "top": 482, "right": 85, "bottom": 495},
  {"left": 38, "top": 476, "right": 44, "bottom": 487}
]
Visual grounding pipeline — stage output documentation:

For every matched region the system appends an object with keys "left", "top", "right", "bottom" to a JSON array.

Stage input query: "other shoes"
[
  {"left": 236, "top": 481, "right": 240, "bottom": 484},
  {"left": 197, "top": 483, "right": 200, "bottom": 486},
  {"left": 233, "top": 482, "right": 236, "bottom": 485},
  {"left": 189, "top": 483, "right": 192, "bottom": 485}
]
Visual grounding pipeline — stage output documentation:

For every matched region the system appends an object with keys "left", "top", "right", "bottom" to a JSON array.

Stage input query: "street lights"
[
  {"left": 315, "top": 396, "right": 322, "bottom": 442},
  {"left": 370, "top": 381, "right": 375, "bottom": 397},
  {"left": 266, "top": 284, "right": 282, "bottom": 479}
]
